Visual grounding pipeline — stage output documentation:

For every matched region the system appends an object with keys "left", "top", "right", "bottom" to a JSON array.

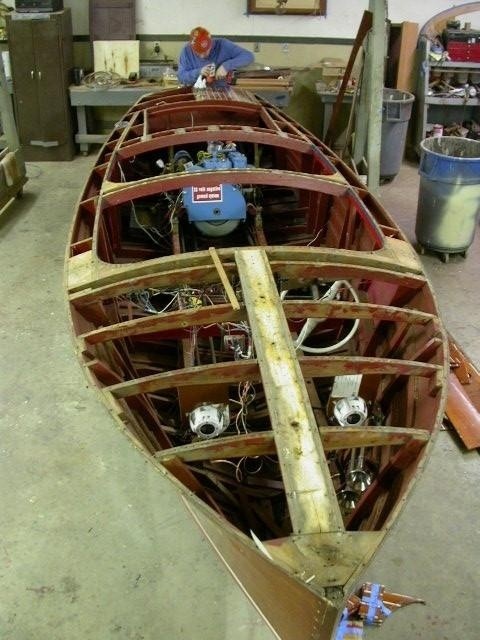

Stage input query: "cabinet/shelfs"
[
  {"left": 4, "top": 7, "right": 79, "bottom": 161},
  {"left": 412, "top": 37, "right": 480, "bottom": 163}
]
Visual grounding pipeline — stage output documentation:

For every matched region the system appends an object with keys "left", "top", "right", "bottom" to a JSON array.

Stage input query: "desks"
[
  {"left": 315, "top": 81, "right": 354, "bottom": 142},
  {"left": 66, "top": 79, "right": 291, "bottom": 156}
]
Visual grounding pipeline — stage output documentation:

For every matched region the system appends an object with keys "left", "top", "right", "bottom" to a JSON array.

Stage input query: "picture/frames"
[{"left": 246, "top": 0, "right": 327, "bottom": 16}]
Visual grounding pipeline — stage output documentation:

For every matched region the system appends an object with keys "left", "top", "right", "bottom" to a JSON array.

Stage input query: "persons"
[{"left": 177, "top": 27, "right": 255, "bottom": 86}]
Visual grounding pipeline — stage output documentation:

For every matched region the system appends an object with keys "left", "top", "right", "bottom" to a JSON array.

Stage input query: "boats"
[{"left": 62, "top": 83, "right": 451, "bottom": 640}]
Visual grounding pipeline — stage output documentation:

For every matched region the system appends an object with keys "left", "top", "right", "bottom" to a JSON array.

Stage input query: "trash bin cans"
[
  {"left": 380, "top": 88, "right": 416, "bottom": 186},
  {"left": 415, "top": 136, "right": 480, "bottom": 263}
]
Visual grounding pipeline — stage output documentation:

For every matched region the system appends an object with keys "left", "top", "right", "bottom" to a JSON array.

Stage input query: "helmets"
[{"left": 188, "top": 26, "right": 213, "bottom": 54}]
